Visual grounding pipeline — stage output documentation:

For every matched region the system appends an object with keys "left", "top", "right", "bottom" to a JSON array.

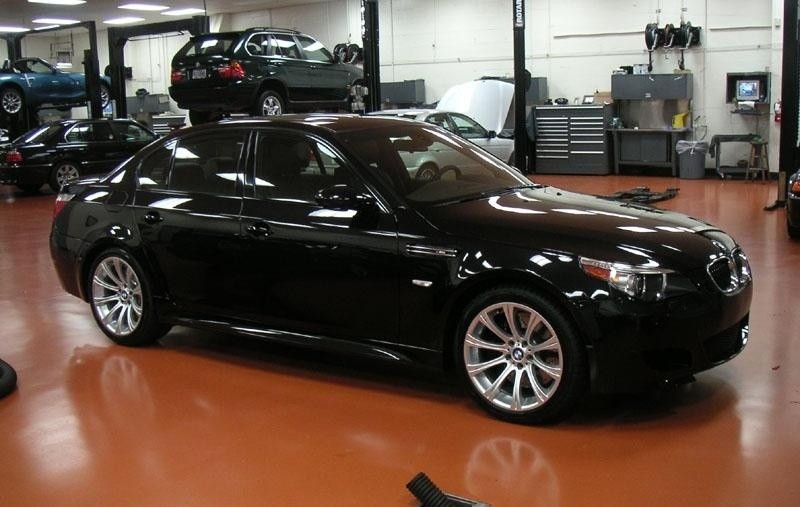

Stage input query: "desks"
[{"left": 608, "top": 127, "right": 689, "bottom": 176}]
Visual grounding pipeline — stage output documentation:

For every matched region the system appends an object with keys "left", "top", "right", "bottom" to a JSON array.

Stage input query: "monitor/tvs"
[{"left": 737, "top": 80, "right": 759, "bottom": 100}]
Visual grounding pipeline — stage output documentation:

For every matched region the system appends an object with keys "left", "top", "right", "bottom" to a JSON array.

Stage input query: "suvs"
[{"left": 168, "top": 23, "right": 366, "bottom": 126}]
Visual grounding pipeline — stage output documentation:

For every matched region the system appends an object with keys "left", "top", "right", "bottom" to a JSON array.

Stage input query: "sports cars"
[{"left": 0, "top": 55, "right": 117, "bottom": 117}]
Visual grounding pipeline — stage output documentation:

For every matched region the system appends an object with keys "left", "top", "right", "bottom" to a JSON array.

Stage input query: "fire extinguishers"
[{"left": 774, "top": 98, "right": 782, "bottom": 121}]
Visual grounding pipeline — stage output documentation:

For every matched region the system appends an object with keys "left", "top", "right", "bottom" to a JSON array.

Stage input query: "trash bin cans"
[{"left": 676, "top": 140, "right": 709, "bottom": 179}]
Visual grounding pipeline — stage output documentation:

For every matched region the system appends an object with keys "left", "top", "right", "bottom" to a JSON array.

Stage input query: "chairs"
[
  {"left": 206, "top": 157, "right": 239, "bottom": 184},
  {"left": 334, "top": 142, "right": 394, "bottom": 202},
  {"left": 166, "top": 163, "right": 206, "bottom": 191},
  {"left": 268, "top": 141, "right": 330, "bottom": 213}
]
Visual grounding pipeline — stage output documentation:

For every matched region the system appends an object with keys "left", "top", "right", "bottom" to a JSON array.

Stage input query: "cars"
[
  {"left": 303, "top": 76, "right": 524, "bottom": 187},
  {"left": 781, "top": 167, "right": 800, "bottom": 245},
  {"left": 44, "top": 111, "right": 761, "bottom": 429},
  {"left": 0, "top": 112, "right": 180, "bottom": 200},
  {"left": 60, "top": 340, "right": 746, "bottom": 506}
]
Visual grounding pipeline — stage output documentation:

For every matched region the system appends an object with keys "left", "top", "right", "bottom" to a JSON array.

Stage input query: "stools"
[{"left": 745, "top": 141, "right": 772, "bottom": 185}]
